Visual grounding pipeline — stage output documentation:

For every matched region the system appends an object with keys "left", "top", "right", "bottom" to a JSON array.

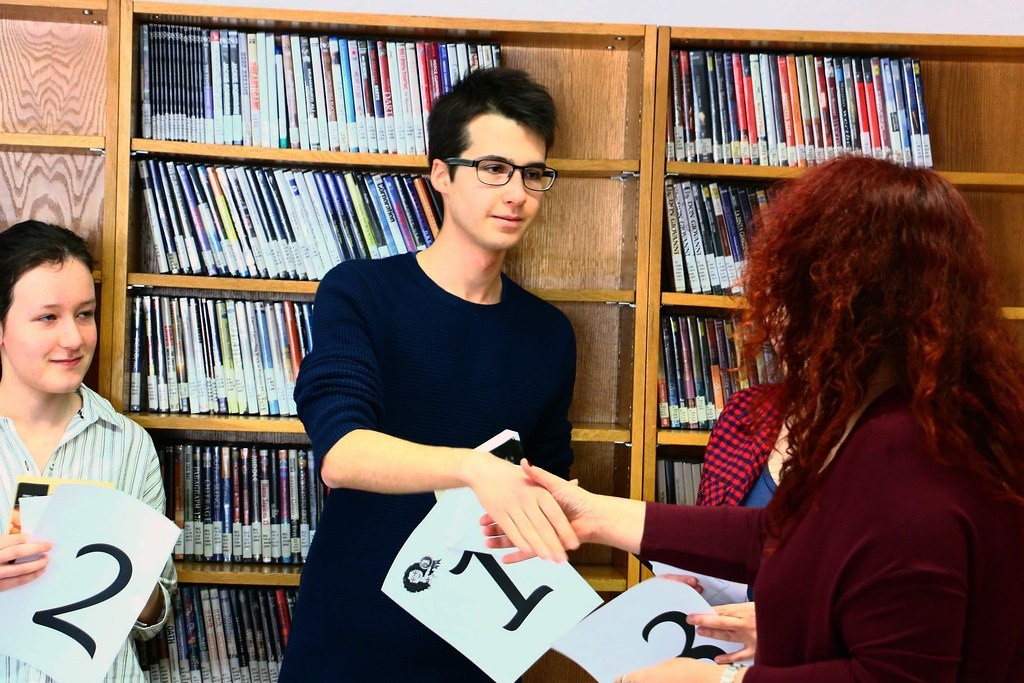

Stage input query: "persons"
[
  {"left": 277, "top": 66, "right": 578, "bottom": 682},
  {"left": 0, "top": 217, "right": 181, "bottom": 682},
  {"left": 482, "top": 154, "right": 1024, "bottom": 681}
]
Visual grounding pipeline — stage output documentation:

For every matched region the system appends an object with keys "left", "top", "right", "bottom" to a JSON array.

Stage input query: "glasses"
[{"left": 445, "top": 158, "right": 558, "bottom": 191}]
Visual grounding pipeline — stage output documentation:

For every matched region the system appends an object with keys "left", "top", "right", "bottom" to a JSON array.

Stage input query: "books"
[
  {"left": 131, "top": 17, "right": 505, "bottom": 682},
  {"left": 654, "top": 44, "right": 937, "bottom": 505},
  {"left": 2, "top": 472, "right": 117, "bottom": 588}
]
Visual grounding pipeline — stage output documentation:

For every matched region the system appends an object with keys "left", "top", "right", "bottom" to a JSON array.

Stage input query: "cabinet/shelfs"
[{"left": 0, "top": 0, "right": 1024, "bottom": 683}]
